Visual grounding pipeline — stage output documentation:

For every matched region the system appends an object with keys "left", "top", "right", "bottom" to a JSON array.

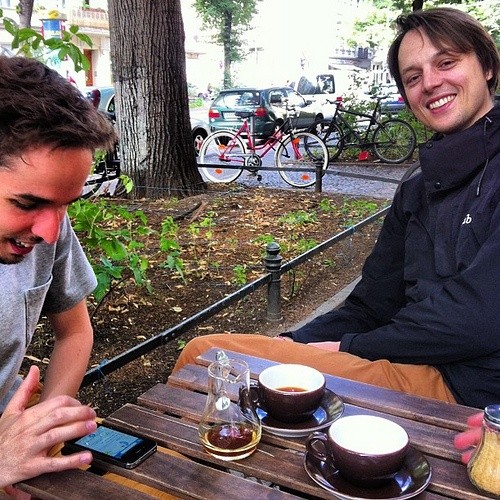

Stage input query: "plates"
[
  {"left": 301, "top": 434, "right": 432, "bottom": 499},
  {"left": 239, "top": 389, "right": 345, "bottom": 437}
]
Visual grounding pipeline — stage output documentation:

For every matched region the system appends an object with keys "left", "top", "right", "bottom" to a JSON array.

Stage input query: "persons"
[
  {"left": 163, "top": 8, "right": 498, "bottom": 428},
  {"left": 1, "top": 52, "right": 116, "bottom": 488}
]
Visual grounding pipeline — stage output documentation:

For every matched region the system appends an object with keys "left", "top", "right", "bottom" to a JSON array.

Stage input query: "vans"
[{"left": 293, "top": 69, "right": 377, "bottom": 131}]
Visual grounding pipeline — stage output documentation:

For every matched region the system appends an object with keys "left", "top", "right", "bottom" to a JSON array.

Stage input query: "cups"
[
  {"left": 306, "top": 415, "right": 408, "bottom": 479},
  {"left": 250, "top": 365, "right": 326, "bottom": 421}
]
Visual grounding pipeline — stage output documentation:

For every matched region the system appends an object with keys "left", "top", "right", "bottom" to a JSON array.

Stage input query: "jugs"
[{"left": 198, "top": 351, "right": 261, "bottom": 461}]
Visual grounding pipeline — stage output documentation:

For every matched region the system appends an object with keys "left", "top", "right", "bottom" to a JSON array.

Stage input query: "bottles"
[{"left": 467, "top": 405, "right": 500, "bottom": 499}]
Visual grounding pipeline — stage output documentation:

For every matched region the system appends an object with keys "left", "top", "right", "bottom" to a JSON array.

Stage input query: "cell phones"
[{"left": 66, "top": 423, "right": 157, "bottom": 470}]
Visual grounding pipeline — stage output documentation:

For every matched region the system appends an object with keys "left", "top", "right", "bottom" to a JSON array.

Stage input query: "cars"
[
  {"left": 379, "top": 93, "right": 407, "bottom": 114},
  {"left": 88, "top": 86, "right": 212, "bottom": 156},
  {"left": 209, "top": 85, "right": 324, "bottom": 146}
]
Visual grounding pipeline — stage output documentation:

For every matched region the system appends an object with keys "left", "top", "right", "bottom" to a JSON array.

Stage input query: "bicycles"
[
  {"left": 303, "top": 94, "right": 417, "bottom": 164},
  {"left": 198, "top": 99, "right": 330, "bottom": 189}
]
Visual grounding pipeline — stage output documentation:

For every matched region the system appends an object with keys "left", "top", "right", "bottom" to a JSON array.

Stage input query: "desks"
[{"left": 0, "top": 347, "right": 500, "bottom": 500}]
